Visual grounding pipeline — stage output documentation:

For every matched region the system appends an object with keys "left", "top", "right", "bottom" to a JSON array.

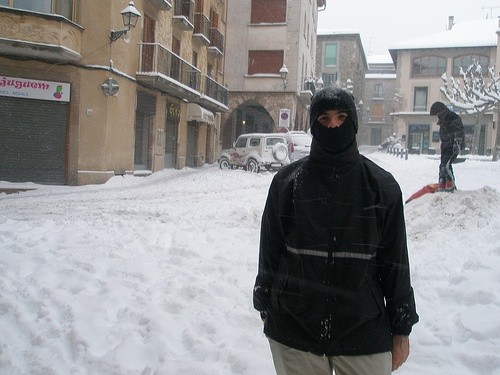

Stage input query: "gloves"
[{"left": 452, "top": 144, "right": 460, "bottom": 154}]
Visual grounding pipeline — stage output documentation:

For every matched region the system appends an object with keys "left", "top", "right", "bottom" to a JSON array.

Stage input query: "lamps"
[
  {"left": 279, "top": 64, "right": 290, "bottom": 88},
  {"left": 111, "top": 1, "right": 141, "bottom": 42}
]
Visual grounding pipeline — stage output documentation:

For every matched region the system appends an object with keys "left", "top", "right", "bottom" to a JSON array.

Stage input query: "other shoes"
[
  {"left": 444, "top": 184, "right": 456, "bottom": 191},
  {"left": 438, "top": 184, "right": 445, "bottom": 192}
]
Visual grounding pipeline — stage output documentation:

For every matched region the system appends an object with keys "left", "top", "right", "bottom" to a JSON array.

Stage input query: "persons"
[
  {"left": 430, "top": 102, "right": 466, "bottom": 192},
  {"left": 253, "top": 88, "right": 419, "bottom": 375}
]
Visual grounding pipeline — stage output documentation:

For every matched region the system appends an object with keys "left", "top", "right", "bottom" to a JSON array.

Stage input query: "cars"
[{"left": 286, "top": 130, "right": 312, "bottom": 163}]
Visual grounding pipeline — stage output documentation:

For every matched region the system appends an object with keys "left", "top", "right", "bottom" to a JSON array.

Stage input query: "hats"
[{"left": 309, "top": 88, "right": 358, "bottom": 133}]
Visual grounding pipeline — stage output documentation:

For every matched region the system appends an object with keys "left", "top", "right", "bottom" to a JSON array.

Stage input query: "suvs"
[{"left": 217, "top": 133, "right": 295, "bottom": 174}]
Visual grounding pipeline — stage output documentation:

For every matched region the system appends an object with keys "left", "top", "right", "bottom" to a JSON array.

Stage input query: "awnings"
[{"left": 187, "top": 102, "right": 215, "bottom": 126}]
